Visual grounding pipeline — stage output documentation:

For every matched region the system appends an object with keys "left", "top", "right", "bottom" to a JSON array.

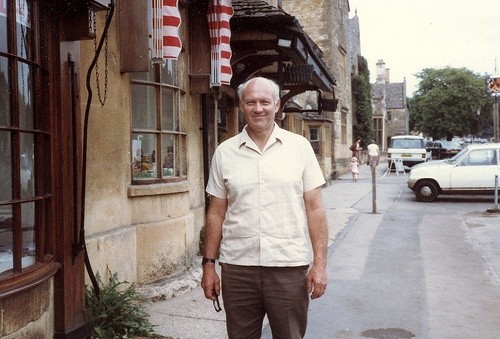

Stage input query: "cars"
[
  {"left": 426, "top": 140, "right": 463, "bottom": 160},
  {"left": 408, "top": 143, "right": 500, "bottom": 202},
  {"left": 452, "top": 137, "right": 488, "bottom": 147}
]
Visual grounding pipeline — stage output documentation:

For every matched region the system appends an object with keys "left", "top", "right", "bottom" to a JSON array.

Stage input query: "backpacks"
[{"left": 350, "top": 142, "right": 358, "bottom": 151}]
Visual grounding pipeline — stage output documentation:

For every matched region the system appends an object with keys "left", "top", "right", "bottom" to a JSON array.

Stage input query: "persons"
[
  {"left": 349, "top": 136, "right": 380, "bottom": 181},
  {"left": 200, "top": 77, "right": 328, "bottom": 339}
]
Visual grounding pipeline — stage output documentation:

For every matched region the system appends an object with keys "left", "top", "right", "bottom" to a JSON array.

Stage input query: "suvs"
[{"left": 387, "top": 134, "right": 427, "bottom": 169}]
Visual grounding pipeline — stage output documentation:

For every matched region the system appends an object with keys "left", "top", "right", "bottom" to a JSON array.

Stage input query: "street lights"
[{"left": 487, "top": 57, "right": 500, "bottom": 143}]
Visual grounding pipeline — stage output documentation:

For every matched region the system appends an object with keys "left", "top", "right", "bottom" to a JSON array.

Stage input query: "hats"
[{"left": 351, "top": 157, "right": 358, "bottom": 161}]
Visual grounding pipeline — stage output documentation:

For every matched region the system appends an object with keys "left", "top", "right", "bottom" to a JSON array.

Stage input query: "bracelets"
[{"left": 202, "top": 257, "right": 215, "bottom": 265}]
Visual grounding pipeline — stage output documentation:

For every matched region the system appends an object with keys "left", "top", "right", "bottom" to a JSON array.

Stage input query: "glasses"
[{"left": 213, "top": 287, "right": 222, "bottom": 313}]
